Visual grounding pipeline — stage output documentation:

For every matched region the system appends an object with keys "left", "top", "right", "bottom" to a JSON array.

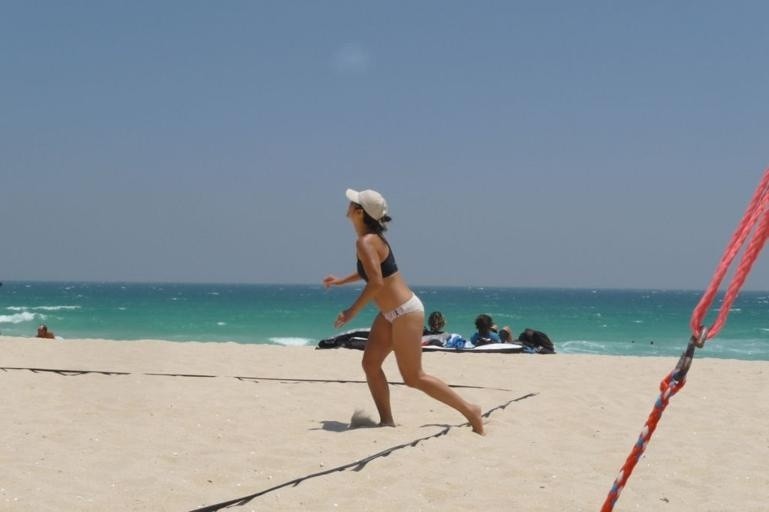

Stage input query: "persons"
[
  {"left": 322, "top": 186, "right": 484, "bottom": 436},
  {"left": 37, "top": 322, "right": 54, "bottom": 338},
  {"left": 422, "top": 307, "right": 446, "bottom": 336},
  {"left": 470, "top": 313, "right": 512, "bottom": 348}
]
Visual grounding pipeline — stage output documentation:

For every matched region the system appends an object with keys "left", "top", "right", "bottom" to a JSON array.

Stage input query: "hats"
[{"left": 346, "top": 189, "right": 387, "bottom": 221}]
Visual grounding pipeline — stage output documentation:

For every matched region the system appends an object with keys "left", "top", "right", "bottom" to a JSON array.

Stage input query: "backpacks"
[{"left": 519, "top": 328, "right": 554, "bottom": 354}]
